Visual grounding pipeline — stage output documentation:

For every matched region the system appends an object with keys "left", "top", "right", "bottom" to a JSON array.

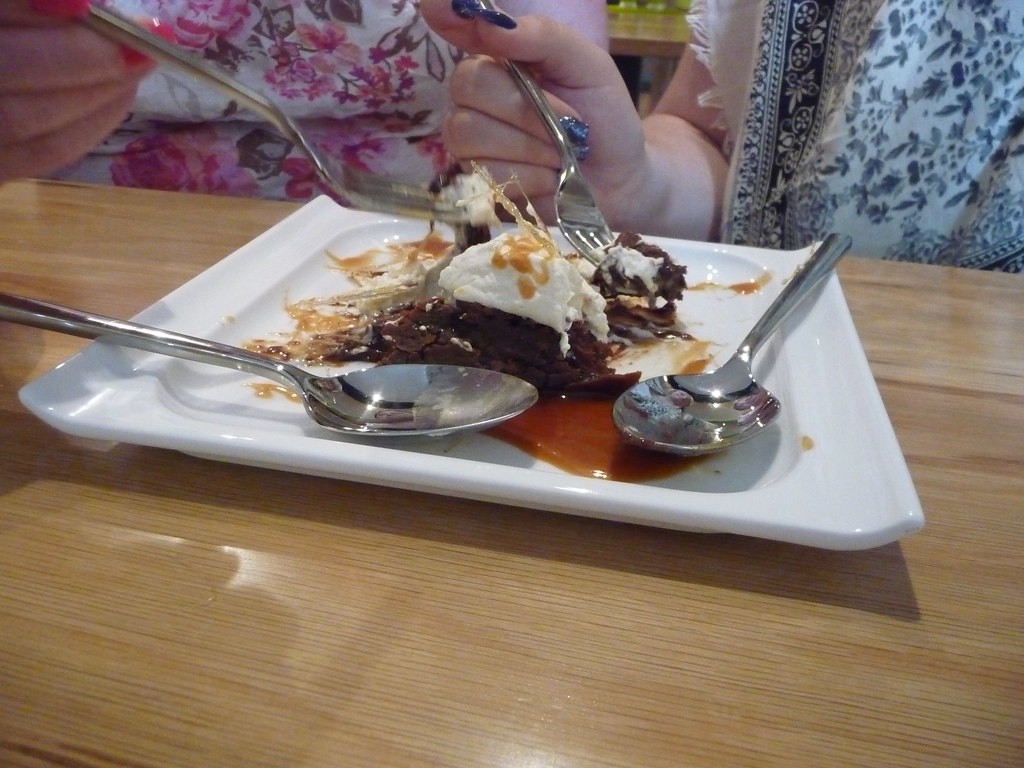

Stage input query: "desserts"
[{"left": 326, "top": 157, "right": 692, "bottom": 398}]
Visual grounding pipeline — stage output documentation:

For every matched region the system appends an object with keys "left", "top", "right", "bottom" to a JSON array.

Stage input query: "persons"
[
  {"left": 447, "top": 0, "right": 1023, "bottom": 272},
  {"left": 0, "top": 0, "right": 609, "bottom": 219}
]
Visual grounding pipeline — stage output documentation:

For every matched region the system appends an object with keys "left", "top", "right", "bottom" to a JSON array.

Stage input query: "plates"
[{"left": 17, "top": 193, "right": 927, "bottom": 549}]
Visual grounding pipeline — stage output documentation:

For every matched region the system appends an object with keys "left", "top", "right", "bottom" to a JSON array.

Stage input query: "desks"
[{"left": 0, "top": 177, "right": 1024, "bottom": 768}]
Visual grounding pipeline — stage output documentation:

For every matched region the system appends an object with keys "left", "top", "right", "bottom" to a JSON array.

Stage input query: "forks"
[
  {"left": 85, "top": 4, "right": 466, "bottom": 222},
  {"left": 477, "top": 0, "right": 617, "bottom": 268}
]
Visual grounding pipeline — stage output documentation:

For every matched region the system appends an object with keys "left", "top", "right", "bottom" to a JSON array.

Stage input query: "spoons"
[
  {"left": 0, "top": 291, "right": 539, "bottom": 438},
  {"left": 612, "top": 232, "right": 854, "bottom": 455}
]
